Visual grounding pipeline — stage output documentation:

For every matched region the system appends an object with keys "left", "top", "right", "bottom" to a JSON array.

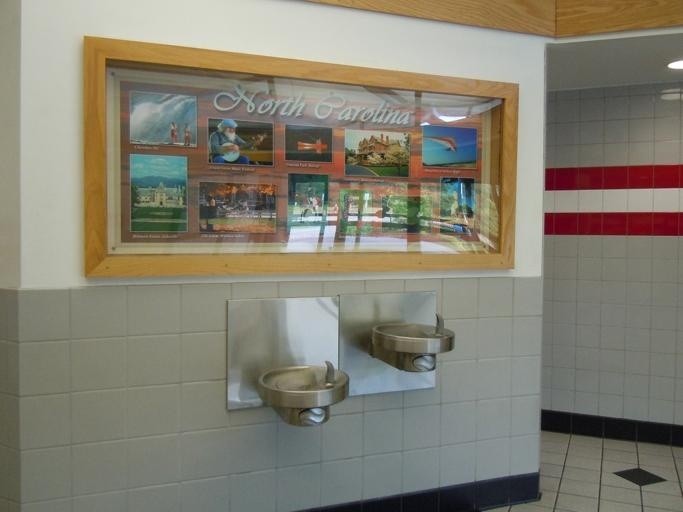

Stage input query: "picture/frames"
[{"left": 84, "top": 35, "right": 519, "bottom": 278}]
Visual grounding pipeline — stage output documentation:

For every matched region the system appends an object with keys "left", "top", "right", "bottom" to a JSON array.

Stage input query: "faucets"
[
  {"left": 325, "top": 360, "right": 337, "bottom": 389},
  {"left": 434, "top": 311, "right": 445, "bottom": 337}
]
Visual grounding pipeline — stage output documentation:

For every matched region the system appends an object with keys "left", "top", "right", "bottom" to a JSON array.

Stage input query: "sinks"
[
  {"left": 257, "top": 365, "right": 350, "bottom": 427},
  {"left": 373, "top": 324, "right": 455, "bottom": 374}
]
